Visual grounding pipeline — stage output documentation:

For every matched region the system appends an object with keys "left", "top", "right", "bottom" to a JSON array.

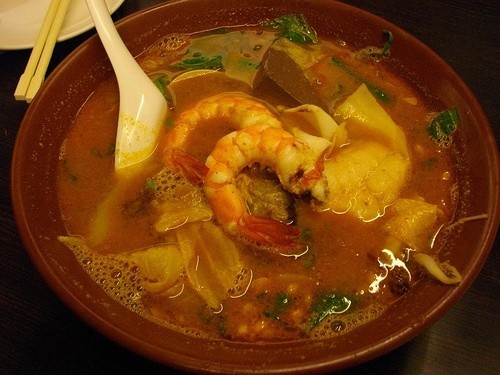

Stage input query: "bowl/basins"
[{"left": 10, "top": 0, "right": 500, "bottom": 374}]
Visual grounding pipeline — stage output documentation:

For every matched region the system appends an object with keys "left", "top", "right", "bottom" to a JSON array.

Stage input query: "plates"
[{"left": 0, "top": 1, "right": 126, "bottom": 49}]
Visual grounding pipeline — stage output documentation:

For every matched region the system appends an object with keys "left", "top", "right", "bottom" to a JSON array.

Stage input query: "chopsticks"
[{"left": 14, "top": 0, "right": 70, "bottom": 103}]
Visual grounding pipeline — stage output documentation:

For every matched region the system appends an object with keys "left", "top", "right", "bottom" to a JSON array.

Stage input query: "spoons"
[{"left": 85, "top": 0, "right": 167, "bottom": 176}]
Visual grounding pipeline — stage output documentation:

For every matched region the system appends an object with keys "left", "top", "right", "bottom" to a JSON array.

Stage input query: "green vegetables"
[{"left": 117, "top": 13, "right": 460, "bottom": 327}]
